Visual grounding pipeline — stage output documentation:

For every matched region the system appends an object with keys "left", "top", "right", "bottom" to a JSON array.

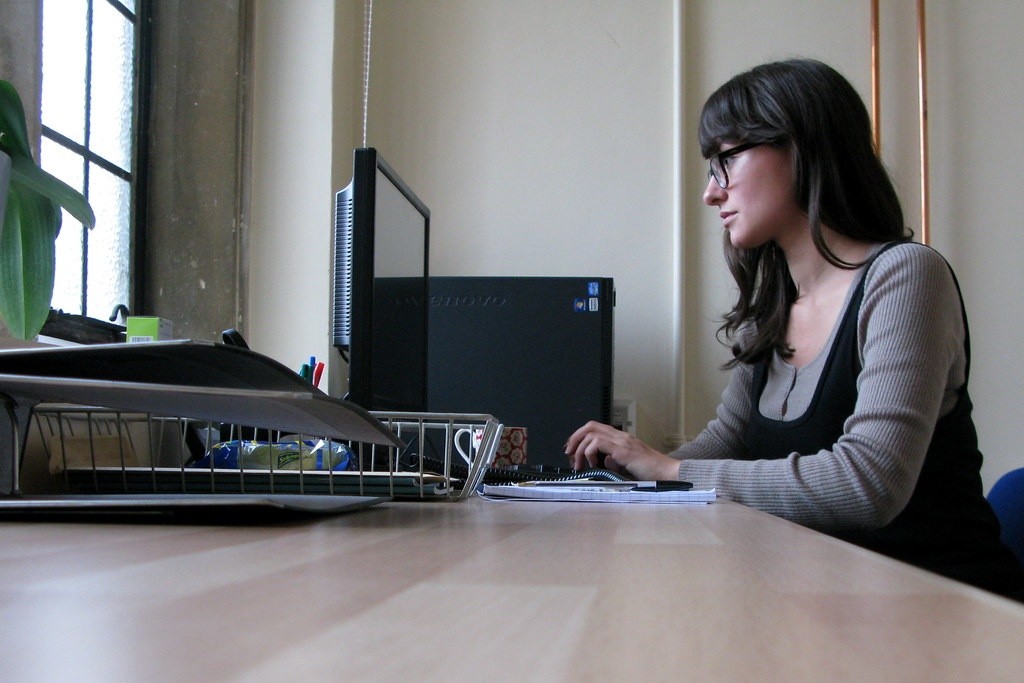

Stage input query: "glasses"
[{"left": 708, "top": 142, "right": 765, "bottom": 189}]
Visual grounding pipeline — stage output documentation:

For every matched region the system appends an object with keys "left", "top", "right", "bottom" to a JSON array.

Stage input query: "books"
[
  {"left": 480, "top": 482, "right": 718, "bottom": 505},
  {"left": 1, "top": 467, "right": 461, "bottom": 519}
]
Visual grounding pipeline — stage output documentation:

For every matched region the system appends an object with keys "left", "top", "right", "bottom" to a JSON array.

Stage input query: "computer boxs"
[{"left": 429, "top": 275, "right": 617, "bottom": 478}]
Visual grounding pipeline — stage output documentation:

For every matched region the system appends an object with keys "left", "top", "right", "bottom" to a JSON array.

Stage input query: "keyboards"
[{"left": 502, "top": 464, "right": 584, "bottom": 477}]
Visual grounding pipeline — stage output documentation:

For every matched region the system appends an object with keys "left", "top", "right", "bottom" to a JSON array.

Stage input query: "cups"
[{"left": 454, "top": 426, "right": 529, "bottom": 470}]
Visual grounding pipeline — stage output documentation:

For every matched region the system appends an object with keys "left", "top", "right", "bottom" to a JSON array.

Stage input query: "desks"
[{"left": 1, "top": 494, "right": 1024, "bottom": 683}]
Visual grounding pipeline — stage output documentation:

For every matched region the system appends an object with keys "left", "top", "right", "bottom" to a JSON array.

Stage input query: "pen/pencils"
[
  {"left": 298, "top": 356, "right": 325, "bottom": 389},
  {"left": 516, "top": 480, "right": 693, "bottom": 491}
]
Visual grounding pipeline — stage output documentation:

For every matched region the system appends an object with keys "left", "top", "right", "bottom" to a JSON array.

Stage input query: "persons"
[{"left": 561, "top": 56, "right": 1020, "bottom": 607}]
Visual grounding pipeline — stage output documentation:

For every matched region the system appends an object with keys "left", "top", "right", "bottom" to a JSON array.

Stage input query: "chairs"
[{"left": 986, "top": 467, "right": 1024, "bottom": 569}]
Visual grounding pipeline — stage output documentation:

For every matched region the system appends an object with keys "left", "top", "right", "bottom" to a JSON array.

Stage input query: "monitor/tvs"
[{"left": 332, "top": 147, "right": 431, "bottom": 470}]
